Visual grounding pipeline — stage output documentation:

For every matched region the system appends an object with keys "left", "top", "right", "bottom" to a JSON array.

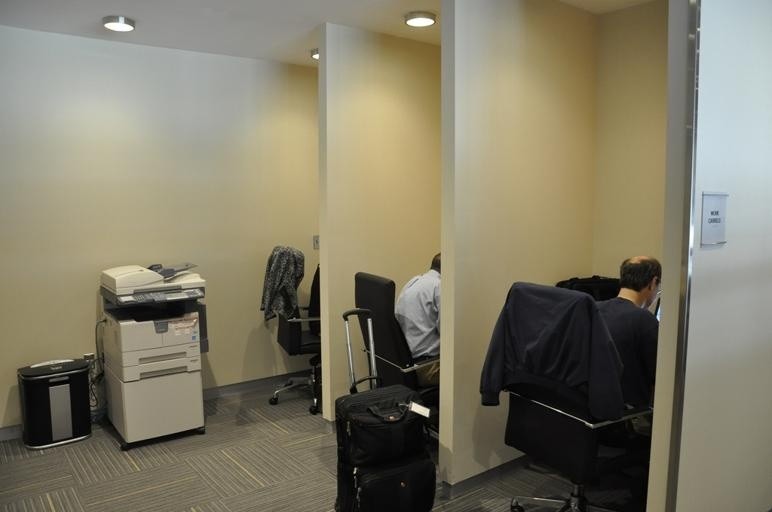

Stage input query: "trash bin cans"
[{"left": 17, "top": 358, "right": 90, "bottom": 450}]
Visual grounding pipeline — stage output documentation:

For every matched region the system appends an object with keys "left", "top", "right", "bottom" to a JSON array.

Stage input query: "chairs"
[
  {"left": 354, "top": 272, "right": 440, "bottom": 441},
  {"left": 503, "top": 282, "right": 654, "bottom": 512},
  {"left": 269, "top": 253, "right": 322, "bottom": 415}
]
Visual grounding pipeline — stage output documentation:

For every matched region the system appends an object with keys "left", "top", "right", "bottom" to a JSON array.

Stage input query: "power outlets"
[{"left": 84, "top": 353, "right": 95, "bottom": 369}]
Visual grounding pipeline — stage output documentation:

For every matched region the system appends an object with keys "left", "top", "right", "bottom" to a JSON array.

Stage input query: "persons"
[
  {"left": 393, "top": 253, "right": 439, "bottom": 388},
  {"left": 308, "top": 265, "right": 320, "bottom": 337},
  {"left": 592, "top": 255, "right": 661, "bottom": 437}
]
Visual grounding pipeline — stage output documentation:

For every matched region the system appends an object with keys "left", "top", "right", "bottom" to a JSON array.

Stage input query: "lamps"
[
  {"left": 311, "top": 49, "right": 320, "bottom": 60},
  {"left": 103, "top": 15, "right": 135, "bottom": 32},
  {"left": 405, "top": 12, "right": 436, "bottom": 28}
]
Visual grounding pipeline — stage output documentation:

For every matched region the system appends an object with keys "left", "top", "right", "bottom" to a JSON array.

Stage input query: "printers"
[{"left": 100, "top": 262, "right": 209, "bottom": 382}]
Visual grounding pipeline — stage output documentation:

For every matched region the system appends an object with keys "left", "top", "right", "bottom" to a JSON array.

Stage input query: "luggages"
[{"left": 335, "top": 309, "right": 437, "bottom": 511}]
